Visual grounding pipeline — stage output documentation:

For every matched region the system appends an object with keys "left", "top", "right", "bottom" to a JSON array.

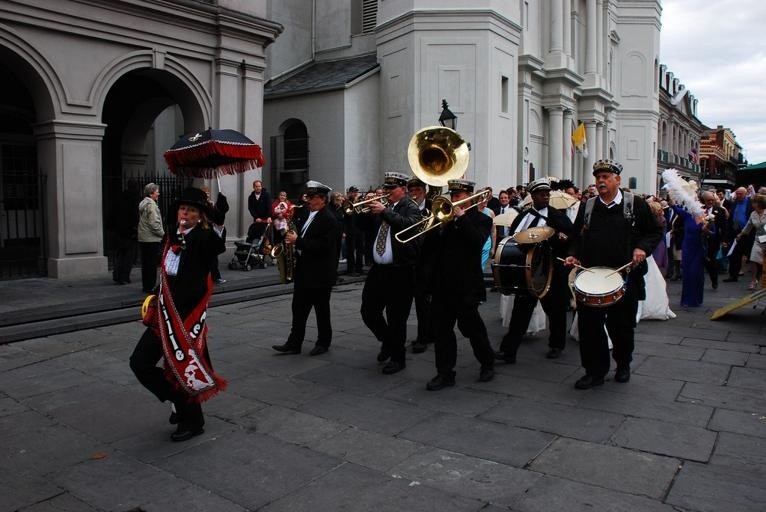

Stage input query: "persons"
[
  {"left": 137, "top": 183, "right": 167, "bottom": 294},
  {"left": 361, "top": 172, "right": 420, "bottom": 374},
  {"left": 299, "top": 194, "right": 308, "bottom": 219},
  {"left": 490, "top": 160, "right": 644, "bottom": 391},
  {"left": 272, "top": 191, "right": 294, "bottom": 243},
  {"left": 272, "top": 180, "right": 342, "bottom": 356},
  {"left": 645, "top": 169, "right": 766, "bottom": 305},
  {"left": 343, "top": 186, "right": 364, "bottom": 275},
  {"left": 363, "top": 192, "right": 379, "bottom": 266},
  {"left": 418, "top": 180, "right": 496, "bottom": 389},
  {"left": 375, "top": 187, "right": 383, "bottom": 203},
  {"left": 475, "top": 191, "right": 495, "bottom": 274},
  {"left": 201, "top": 185, "right": 229, "bottom": 284},
  {"left": 330, "top": 193, "right": 345, "bottom": 283},
  {"left": 262, "top": 237, "right": 272, "bottom": 266},
  {"left": 408, "top": 180, "right": 433, "bottom": 353},
  {"left": 249, "top": 180, "right": 275, "bottom": 245},
  {"left": 129, "top": 188, "right": 228, "bottom": 442},
  {"left": 275, "top": 202, "right": 288, "bottom": 230}
]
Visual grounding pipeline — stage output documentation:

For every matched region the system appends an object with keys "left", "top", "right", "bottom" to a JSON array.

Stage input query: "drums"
[
  {"left": 573, "top": 265, "right": 624, "bottom": 308},
  {"left": 493, "top": 236, "right": 553, "bottom": 299}
]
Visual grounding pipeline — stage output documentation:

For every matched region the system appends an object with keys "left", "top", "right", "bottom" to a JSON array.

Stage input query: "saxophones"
[{"left": 271, "top": 203, "right": 309, "bottom": 284}]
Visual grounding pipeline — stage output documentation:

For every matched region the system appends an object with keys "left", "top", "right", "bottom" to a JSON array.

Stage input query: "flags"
[
  {"left": 572, "top": 124, "right": 588, "bottom": 159},
  {"left": 688, "top": 147, "right": 697, "bottom": 163}
]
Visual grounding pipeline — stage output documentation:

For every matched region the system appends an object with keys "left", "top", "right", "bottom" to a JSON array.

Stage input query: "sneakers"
[
  {"left": 347, "top": 272, "right": 361, "bottom": 277},
  {"left": 143, "top": 287, "right": 156, "bottom": 294},
  {"left": 215, "top": 279, "right": 227, "bottom": 284}
]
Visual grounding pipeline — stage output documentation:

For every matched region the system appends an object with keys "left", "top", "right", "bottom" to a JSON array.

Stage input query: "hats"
[
  {"left": 593, "top": 159, "right": 623, "bottom": 175},
  {"left": 445, "top": 179, "right": 476, "bottom": 194},
  {"left": 303, "top": 180, "right": 332, "bottom": 197},
  {"left": 382, "top": 171, "right": 410, "bottom": 187},
  {"left": 173, "top": 187, "right": 209, "bottom": 213},
  {"left": 407, "top": 176, "right": 426, "bottom": 189},
  {"left": 349, "top": 186, "right": 359, "bottom": 192},
  {"left": 526, "top": 177, "right": 552, "bottom": 195}
]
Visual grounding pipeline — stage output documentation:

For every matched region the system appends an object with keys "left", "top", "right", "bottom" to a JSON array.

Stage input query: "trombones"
[{"left": 394, "top": 189, "right": 490, "bottom": 244}]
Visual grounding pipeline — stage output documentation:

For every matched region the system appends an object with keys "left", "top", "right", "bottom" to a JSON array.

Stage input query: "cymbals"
[{"left": 513, "top": 225, "right": 554, "bottom": 244}]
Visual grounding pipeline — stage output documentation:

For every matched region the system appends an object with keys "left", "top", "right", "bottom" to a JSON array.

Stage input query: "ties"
[
  {"left": 569, "top": 207, "right": 573, "bottom": 220},
  {"left": 527, "top": 216, "right": 539, "bottom": 229},
  {"left": 499, "top": 208, "right": 504, "bottom": 236},
  {"left": 301, "top": 218, "right": 313, "bottom": 236},
  {"left": 732, "top": 204, "right": 742, "bottom": 230},
  {"left": 376, "top": 203, "right": 394, "bottom": 257},
  {"left": 667, "top": 208, "right": 672, "bottom": 222},
  {"left": 704, "top": 208, "right": 709, "bottom": 216}
]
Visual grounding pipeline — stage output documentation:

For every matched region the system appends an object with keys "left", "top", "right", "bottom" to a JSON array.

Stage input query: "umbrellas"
[{"left": 166, "top": 126, "right": 263, "bottom": 193}]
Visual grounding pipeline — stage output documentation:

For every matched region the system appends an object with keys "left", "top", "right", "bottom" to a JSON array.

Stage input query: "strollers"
[{"left": 226, "top": 219, "right": 271, "bottom": 271}]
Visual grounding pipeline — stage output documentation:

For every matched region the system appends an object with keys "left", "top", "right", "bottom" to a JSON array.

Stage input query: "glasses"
[
  {"left": 383, "top": 186, "right": 402, "bottom": 190},
  {"left": 596, "top": 172, "right": 613, "bottom": 179},
  {"left": 538, "top": 193, "right": 551, "bottom": 197},
  {"left": 305, "top": 195, "right": 322, "bottom": 199}
]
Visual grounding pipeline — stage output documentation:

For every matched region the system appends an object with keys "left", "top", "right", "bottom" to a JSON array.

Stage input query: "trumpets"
[{"left": 342, "top": 193, "right": 390, "bottom": 215}]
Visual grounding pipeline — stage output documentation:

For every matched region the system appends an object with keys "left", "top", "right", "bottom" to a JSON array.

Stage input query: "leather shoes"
[
  {"left": 114, "top": 278, "right": 128, "bottom": 285},
  {"left": 712, "top": 283, "right": 718, "bottom": 290},
  {"left": 171, "top": 424, "right": 204, "bottom": 441},
  {"left": 272, "top": 341, "right": 301, "bottom": 354},
  {"left": 723, "top": 277, "right": 738, "bottom": 282},
  {"left": 169, "top": 412, "right": 178, "bottom": 425},
  {"left": 545, "top": 351, "right": 561, "bottom": 359},
  {"left": 412, "top": 343, "right": 429, "bottom": 352},
  {"left": 615, "top": 366, "right": 630, "bottom": 383},
  {"left": 310, "top": 344, "right": 328, "bottom": 354},
  {"left": 382, "top": 360, "right": 406, "bottom": 374},
  {"left": 426, "top": 375, "right": 455, "bottom": 390},
  {"left": 479, "top": 367, "right": 495, "bottom": 381},
  {"left": 377, "top": 346, "right": 391, "bottom": 361},
  {"left": 495, "top": 350, "right": 513, "bottom": 363},
  {"left": 575, "top": 373, "right": 604, "bottom": 390}
]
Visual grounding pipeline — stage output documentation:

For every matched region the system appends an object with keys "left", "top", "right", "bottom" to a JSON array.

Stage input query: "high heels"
[{"left": 670, "top": 275, "right": 682, "bottom": 281}]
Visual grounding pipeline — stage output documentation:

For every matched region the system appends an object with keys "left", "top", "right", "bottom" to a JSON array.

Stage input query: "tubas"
[{"left": 407, "top": 126, "right": 469, "bottom": 242}]
